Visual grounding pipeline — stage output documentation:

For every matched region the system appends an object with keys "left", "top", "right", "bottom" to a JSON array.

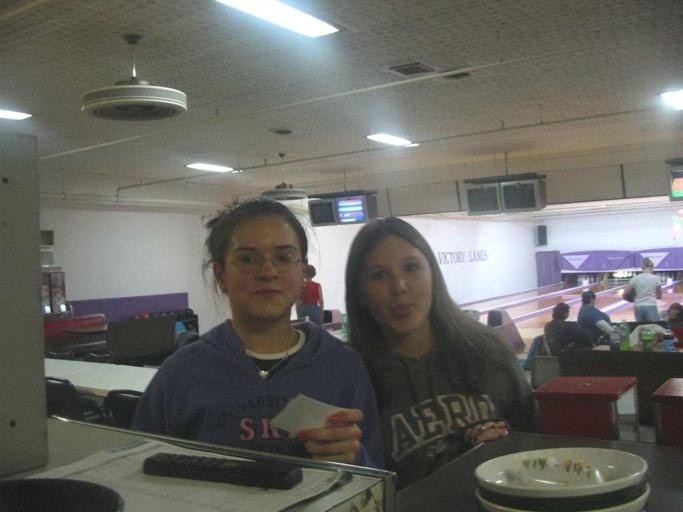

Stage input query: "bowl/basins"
[
  {"left": 475, "top": 447, "right": 648, "bottom": 512},
  {"left": 475, "top": 484, "right": 650, "bottom": 512}
]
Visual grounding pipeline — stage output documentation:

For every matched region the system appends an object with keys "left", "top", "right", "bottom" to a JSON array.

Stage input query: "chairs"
[
  {"left": 44, "top": 375, "right": 116, "bottom": 425},
  {"left": 106, "top": 388, "right": 145, "bottom": 430},
  {"left": 531, "top": 355, "right": 561, "bottom": 390}
]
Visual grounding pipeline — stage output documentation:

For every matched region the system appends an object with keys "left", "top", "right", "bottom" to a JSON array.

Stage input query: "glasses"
[{"left": 223, "top": 252, "right": 302, "bottom": 273}]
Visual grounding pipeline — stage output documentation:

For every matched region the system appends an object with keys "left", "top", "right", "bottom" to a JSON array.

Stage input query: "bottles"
[
  {"left": 662, "top": 325, "right": 674, "bottom": 352},
  {"left": 609, "top": 326, "right": 621, "bottom": 353},
  {"left": 641, "top": 326, "right": 653, "bottom": 351},
  {"left": 618, "top": 319, "right": 629, "bottom": 352}
]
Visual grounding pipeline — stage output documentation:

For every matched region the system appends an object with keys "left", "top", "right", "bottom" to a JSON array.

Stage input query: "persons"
[
  {"left": 339, "top": 217, "right": 540, "bottom": 489},
  {"left": 622, "top": 257, "right": 664, "bottom": 322},
  {"left": 129, "top": 196, "right": 385, "bottom": 471},
  {"left": 578, "top": 291, "right": 617, "bottom": 343},
  {"left": 543, "top": 303, "right": 587, "bottom": 355},
  {"left": 296, "top": 265, "right": 324, "bottom": 325}
]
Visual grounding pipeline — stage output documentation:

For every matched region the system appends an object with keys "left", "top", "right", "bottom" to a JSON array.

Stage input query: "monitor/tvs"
[
  {"left": 308, "top": 197, "right": 339, "bottom": 227},
  {"left": 334, "top": 194, "right": 377, "bottom": 226},
  {"left": 464, "top": 183, "right": 503, "bottom": 216},
  {"left": 498, "top": 179, "right": 546, "bottom": 213},
  {"left": 668, "top": 166, "right": 683, "bottom": 201}
]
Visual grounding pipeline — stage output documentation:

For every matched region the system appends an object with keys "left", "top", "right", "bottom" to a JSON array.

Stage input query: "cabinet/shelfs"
[
  {"left": 651, "top": 378, "right": 683, "bottom": 447},
  {"left": 532, "top": 377, "right": 639, "bottom": 441}
]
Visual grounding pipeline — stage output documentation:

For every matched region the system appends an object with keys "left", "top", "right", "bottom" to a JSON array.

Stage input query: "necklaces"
[{"left": 242, "top": 338, "right": 299, "bottom": 379}]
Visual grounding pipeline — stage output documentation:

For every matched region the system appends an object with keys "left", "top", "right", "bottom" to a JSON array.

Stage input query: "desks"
[
  {"left": 393, "top": 432, "right": 683, "bottom": 512},
  {"left": 43, "top": 357, "right": 159, "bottom": 403}
]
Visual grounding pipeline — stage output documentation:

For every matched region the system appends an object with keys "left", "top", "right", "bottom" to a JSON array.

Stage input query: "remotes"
[{"left": 143, "top": 453, "right": 303, "bottom": 489}]
